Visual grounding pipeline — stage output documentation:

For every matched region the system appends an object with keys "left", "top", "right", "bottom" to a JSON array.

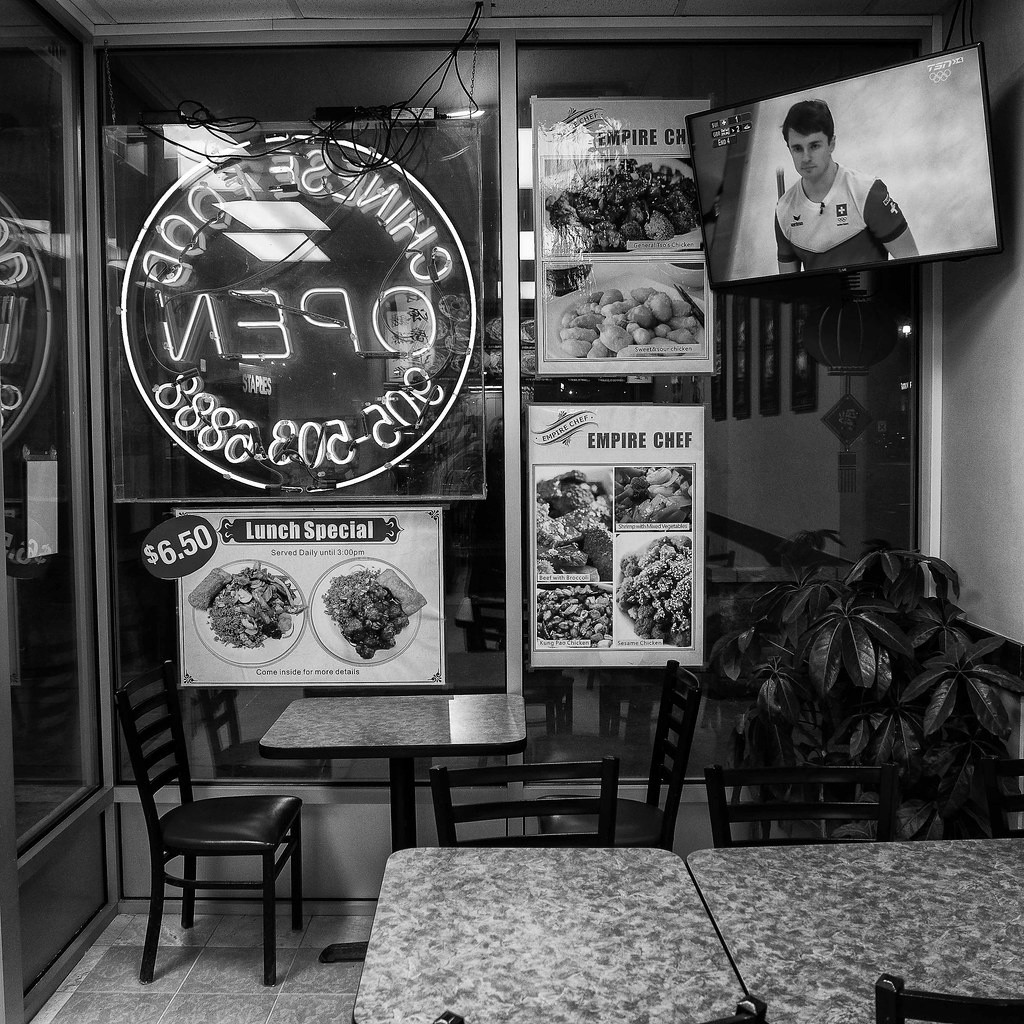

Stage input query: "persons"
[{"left": 772, "top": 98, "right": 921, "bottom": 275}]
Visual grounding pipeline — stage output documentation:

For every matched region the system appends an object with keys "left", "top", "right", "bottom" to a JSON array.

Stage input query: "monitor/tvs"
[{"left": 685, "top": 42, "right": 1002, "bottom": 290}]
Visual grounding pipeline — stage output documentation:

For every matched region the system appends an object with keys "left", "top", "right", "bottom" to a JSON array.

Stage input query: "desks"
[
  {"left": 261, "top": 693, "right": 528, "bottom": 964},
  {"left": 685, "top": 835, "right": 1023, "bottom": 1024},
  {"left": 350, "top": 846, "right": 762, "bottom": 1024}
]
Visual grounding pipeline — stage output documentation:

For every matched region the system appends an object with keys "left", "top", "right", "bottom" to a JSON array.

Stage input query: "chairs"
[
  {"left": 702, "top": 763, "right": 900, "bottom": 848},
  {"left": 873, "top": 973, "right": 1022, "bottom": 1024},
  {"left": 978, "top": 758, "right": 1024, "bottom": 838},
  {"left": 535, "top": 658, "right": 703, "bottom": 849},
  {"left": 115, "top": 660, "right": 305, "bottom": 987},
  {"left": 430, "top": 754, "right": 619, "bottom": 849}
]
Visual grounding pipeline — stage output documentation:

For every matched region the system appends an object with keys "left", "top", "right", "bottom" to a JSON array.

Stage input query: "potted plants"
[{"left": 697, "top": 526, "right": 1023, "bottom": 844}]
